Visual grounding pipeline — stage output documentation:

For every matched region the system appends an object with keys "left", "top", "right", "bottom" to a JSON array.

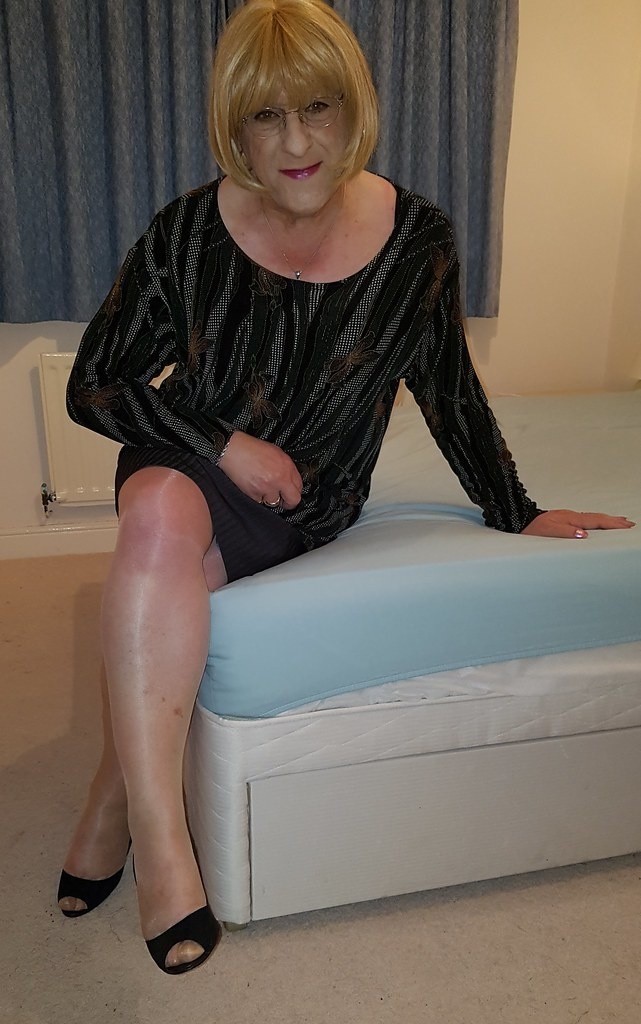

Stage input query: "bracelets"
[{"left": 215, "top": 439, "right": 230, "bottom": 469}]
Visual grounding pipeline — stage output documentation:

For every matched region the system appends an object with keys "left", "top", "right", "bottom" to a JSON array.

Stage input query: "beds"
[{"left": 180, "top": 389, "right": 641, "bottom": 933}]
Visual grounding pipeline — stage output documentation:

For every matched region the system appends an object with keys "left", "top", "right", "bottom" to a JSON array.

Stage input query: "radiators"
[{"left": 37, "top": 353, "right": 127, "bottom": 518}]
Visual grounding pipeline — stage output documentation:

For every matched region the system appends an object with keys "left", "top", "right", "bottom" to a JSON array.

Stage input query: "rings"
[{"left": 257, "top": 497, "right": 280, "bottom": 507}]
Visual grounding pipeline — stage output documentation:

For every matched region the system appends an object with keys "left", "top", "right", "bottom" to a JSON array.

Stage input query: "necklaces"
[{"left": 260, "top": 176, "right": 347, "bottom": 281}]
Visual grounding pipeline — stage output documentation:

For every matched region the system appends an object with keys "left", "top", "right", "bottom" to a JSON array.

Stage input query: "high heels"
[
  {"left": 132, "top": 809, "right": 222, "bottom": 977},
  {"left": 56, "top": 858, "right": 126, "bottom": 917}
]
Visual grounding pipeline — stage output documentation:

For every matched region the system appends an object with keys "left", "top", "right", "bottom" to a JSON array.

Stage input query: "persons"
[{"left": 56, "top": 0, "right": 636, "bottom": 976}]
